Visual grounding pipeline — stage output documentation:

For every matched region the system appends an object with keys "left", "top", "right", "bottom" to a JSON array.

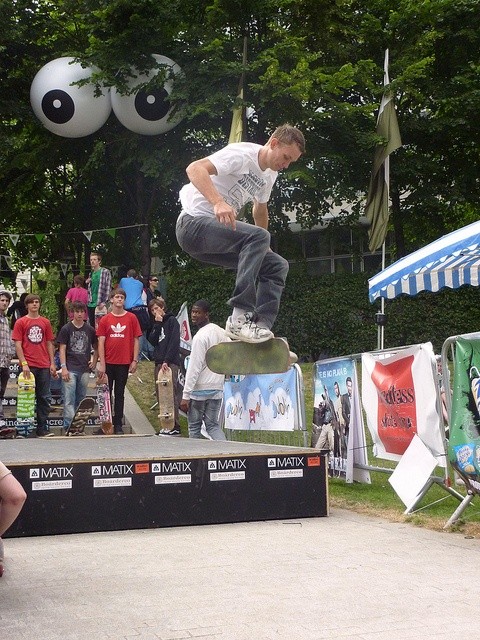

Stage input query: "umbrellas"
[{"left": 367, "top": 220, "right": 480, "bottom": 305}]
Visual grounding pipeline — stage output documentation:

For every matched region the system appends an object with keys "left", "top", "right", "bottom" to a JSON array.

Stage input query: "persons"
[
  {"left": 11, "top": 293, "right": 56, "bottom": 439},
  {"left": 174, "top": 122, "right": 305, "bottom": 363},
  {"left": 180, "top": 300, "right": 232, "bottom": 441},
  {"left": 314, "top": 385, "right": 334, "bottom": 456},
  {"left": 138, "top": 275, "right": 155, "bottom": 360},
  {"left": 64, "top": 275, "right": 88, "bottom": 325},
  {"left": 146, "top": 299, "right": 181, "bottom": 437},
  {"left": 0, "top": 291, "right": 12, "bottom": 431},
  {"left": 147, "top": 276, "right": 161, "bottom": 302},
  {"left": 334, "top": 381, "right": 345, "bottom": 457},
  {"left": 0, "top": 461, "right": 27, "bottom": 576},
  {"left": 84, "top": 252, "right": 111, "bottom": 354},
  {"left": 93, "top": 287, "right": 142, "bottom": 435},
  {"left": 6, "top": 293, "right": 29, "bottom": 330},
  {"left": 341, "top": 377, "right": 352, "bottom": 436},
  {"left": 117, "top": 268, "right": 144, "bottom": 362},
  {"left": 56, "top": 299, "right": 99, "bottom": 435}
]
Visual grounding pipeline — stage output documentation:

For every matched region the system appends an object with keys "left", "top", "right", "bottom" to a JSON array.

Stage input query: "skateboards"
[
  {"left": 95, "top": 305, "right": 107, "bottom": 334},
  {"left": 155, "top": 367, "right": 174, "bottom": 430},
  {"left": 65, "top": 397, "right": 95, "bottom": 436},
  {"left": 205, "top": 337, "right": 298, "bottom": 375},
  {"left": 0, "top": 428, "right": 16, "bottom": 439},
  {"left": 96, "top": 373, "right": 114, "bottom": 434},
  {"left": 16, "top": 371, "right": 36, "bottom": 437}
]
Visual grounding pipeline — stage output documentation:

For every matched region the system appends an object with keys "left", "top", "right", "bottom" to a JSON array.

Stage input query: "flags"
[{"left": 365, "top": 49, "right": 403, "bottom": 255}]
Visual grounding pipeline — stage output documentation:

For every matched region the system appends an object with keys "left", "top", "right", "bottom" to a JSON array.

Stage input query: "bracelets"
[
  {"left": 22, "top": 361, "right": 28, "bottom": 366},
  {"left": 60, "top": 363, "right": 66, "bottom": 367},
  {"left": 133, "top": 360, "right": 139, "bottom": 363}
]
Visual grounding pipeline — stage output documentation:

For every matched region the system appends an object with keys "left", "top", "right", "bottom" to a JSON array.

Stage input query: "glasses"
[{"left": 150, "top": 279, "right": 159, "bottom": 281}]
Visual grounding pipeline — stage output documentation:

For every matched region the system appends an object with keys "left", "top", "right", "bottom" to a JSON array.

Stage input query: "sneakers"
[
  {"left": 160, "top": 427, "right": 182, "bottom": 437},
  {"left": 114, "top": 425, "right": 124, "bottom": 434},
  {"left": 224, "top": 312, "right": 274, "bottom": 344},
  {"left": 155, "top": 428, "right": 166, "bottom": 437},
  {"left": 37, "top": 430, "right": 56, "bottom": 437},
  {"left": 16, "top": 433, "right": 27, "bottom": 439}
]
